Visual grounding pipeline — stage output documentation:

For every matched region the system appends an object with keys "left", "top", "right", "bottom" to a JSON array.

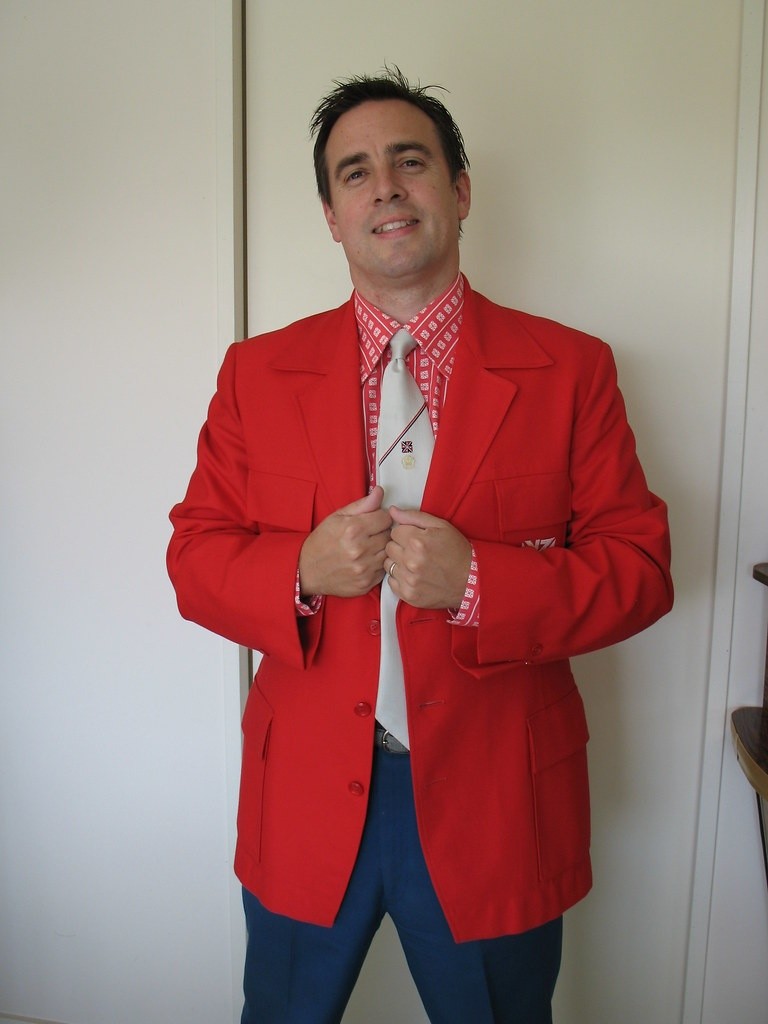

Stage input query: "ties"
[{"left": 374, "top": 329, "right": 437, "bottom": 752}]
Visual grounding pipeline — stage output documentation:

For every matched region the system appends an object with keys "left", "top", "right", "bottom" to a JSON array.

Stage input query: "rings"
[{"left": 389, "top": 562, "right": 397, "bottom": 577}]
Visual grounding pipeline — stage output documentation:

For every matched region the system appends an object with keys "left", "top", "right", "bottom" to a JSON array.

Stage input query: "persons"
[{"left": 165, "top": 65, "right": 674, "bottom": 1024}]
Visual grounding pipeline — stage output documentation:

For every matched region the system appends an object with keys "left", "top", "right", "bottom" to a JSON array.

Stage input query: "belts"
[{"left": 374, "top": 728, "right": 411, "bottom": 753}]
